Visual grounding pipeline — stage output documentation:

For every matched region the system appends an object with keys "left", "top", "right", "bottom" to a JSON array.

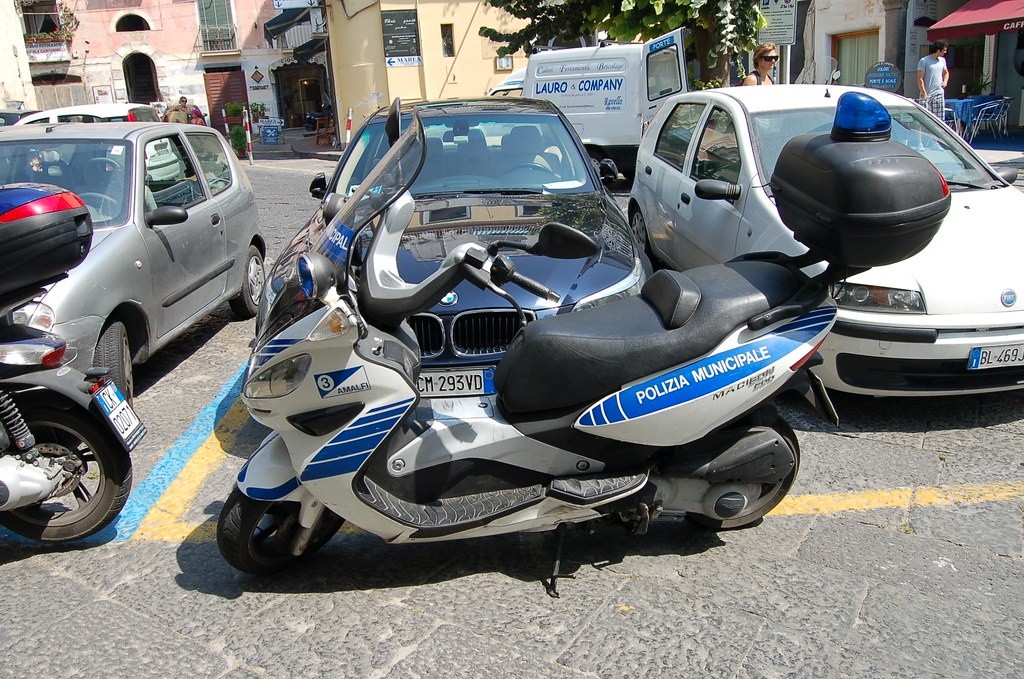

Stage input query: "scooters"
[{"left": 212, "top": 90, "right": 951, "bottom": 583}]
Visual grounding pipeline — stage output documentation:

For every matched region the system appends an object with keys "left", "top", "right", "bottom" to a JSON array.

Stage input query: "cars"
[
  {"left": 255, "top": 96, "right": 654, "bottom": 399},
  {"left": 625, "top": 82, "right": 1024, "bottom": 398},
  {"left": 0, "top": 100, "right": 185, "bottom": 189}
]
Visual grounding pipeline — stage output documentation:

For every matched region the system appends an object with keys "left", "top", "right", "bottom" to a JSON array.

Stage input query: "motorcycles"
[{"left": 0, "top": 180, "right": 147, "bottom": 547}]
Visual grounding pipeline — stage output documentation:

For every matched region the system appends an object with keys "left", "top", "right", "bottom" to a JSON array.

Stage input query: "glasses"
[
  {"left": 182, "top": 101, "right": 187, "bottom": 102},
  {"left": 759, "top": 53, "right": 779, "bottom": 61}
]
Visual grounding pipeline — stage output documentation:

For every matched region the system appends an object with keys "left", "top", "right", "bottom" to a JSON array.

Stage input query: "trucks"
[{"left": 489, "top": 23, "right": 689, "bottom": 185}]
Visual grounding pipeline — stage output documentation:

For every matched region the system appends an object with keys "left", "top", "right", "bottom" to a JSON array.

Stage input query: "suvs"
[{"left": 0, "top": 125, "right": 268, "bottom": 405}]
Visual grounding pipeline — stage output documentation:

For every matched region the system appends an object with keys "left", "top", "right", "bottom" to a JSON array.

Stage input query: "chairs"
[
  {"left": 945, "top": 95, "right": 1014, "bottom": 144},
  {"left": 42, "top": 157, "right": 157, "bottom": 217},
  {"left": 399, "top": 125, "right": 547, "bottom": 182}
]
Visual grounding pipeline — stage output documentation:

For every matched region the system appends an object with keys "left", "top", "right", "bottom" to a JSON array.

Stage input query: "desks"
[
  {"left": 944, "top": 98, "right": 974, "bottom": 140},
  {"left": 969, "top": 95, "right": 1008, "bottom": 130}
]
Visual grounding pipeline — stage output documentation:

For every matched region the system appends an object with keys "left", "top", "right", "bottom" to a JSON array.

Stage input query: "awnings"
[{"left": 926, "top": 0, "right": 1024, "bottom": 42}]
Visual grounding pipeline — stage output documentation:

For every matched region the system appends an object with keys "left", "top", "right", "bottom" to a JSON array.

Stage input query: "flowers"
[{"left": 50, "top": 29, "right": 64, "bottom": 36}]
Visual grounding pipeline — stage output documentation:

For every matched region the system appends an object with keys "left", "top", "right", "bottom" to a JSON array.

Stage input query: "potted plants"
[
  {"left": 24, "top": 29, "right": 33, "bottom": 43},
  {"left": 230, "top": 125, "right": 247, "bottom": 157},
  {"left": 65, "top": 31, "right": 75, "bottom": 41},
  {"left": 34, "top": 32, "right": 52, "bottom": 43},
  {"left": 225, "top": 101, "right": 246, "bottom": 123},
  {"left": 250, "top": 103, "right": 269, "bottom": 122}
]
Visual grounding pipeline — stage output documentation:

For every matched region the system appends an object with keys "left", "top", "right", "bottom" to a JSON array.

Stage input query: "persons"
[
  {"left": 917, "top": 39, "right": 949, "bottom": 122},
  {"left": 177, "top": 97, "right": 207, "bottom": 126},
  {"left": 742, "top": 43, "right": 778, "bottom": 85}
]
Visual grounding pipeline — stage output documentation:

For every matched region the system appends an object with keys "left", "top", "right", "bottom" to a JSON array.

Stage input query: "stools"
[{"left": 315, "top": 118, "right": 332, "bottom": 145}]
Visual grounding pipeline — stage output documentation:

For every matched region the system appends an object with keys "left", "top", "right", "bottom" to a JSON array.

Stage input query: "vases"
[{"left": 52, "top": 36, "right": 64, "bottom": 41}]
